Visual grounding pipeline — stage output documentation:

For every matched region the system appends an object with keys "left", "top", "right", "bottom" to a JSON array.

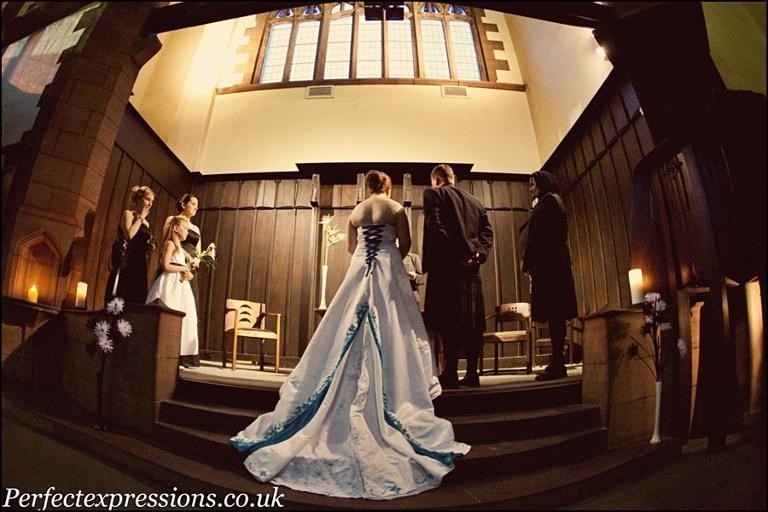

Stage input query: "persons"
[
  {"left": 156, "top": 192, "right": 201, "bottom": 366},
  {"left": 520, "top": 171, "right": 577, "bottom": 381},
  {"left": 145, "top": 216, "right": 200, "bottom": 356},
  {"left": 423, "top": 163, "right": 492, "bottom": 388},
  {"left": 230, "top": 169, "right": 471, "bottom": 500},
  {"left": 105, "top": 186, "right": 155, "bottom": 307}
]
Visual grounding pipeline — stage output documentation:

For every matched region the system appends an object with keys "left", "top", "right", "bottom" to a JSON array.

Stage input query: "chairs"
[
  {"left": 532, "top": 302, "right": 576, "bottom": 367},
  {"left": 221, "top": 296, "right": 282, "bottom": 374},
  {"left": 480, "top": 303, "right": 531, "bottom": 374}
]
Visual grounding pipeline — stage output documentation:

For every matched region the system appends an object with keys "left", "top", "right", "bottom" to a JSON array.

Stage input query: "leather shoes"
[
  {"left": 437, "top": 373, "right": 460, "bottom": 389},
  {"left": 460, "top": 373, "right": 480, "bottom": 387},
  {"left": 535, "top": 364, "right": 568, "bottom": 381}
]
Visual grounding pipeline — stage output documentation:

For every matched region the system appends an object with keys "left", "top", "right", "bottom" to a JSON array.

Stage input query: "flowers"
[
  {"left": 317, "top": 212, "right": 347, "bottom": 265},
  {"left": 84, "top": 296, "right": 133, "bottom": 372},
  {"left": 607, "top": 296, "right": 697, "bottom": 381}
]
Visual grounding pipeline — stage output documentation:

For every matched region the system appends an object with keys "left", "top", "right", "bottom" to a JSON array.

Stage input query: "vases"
[
  {"left": 89, "top": 373, "right": 109, "bottom": 432},
  {"left": 650, "top": 382, "right": 666, "bottom": 444},
  {"left": 319, "top": 265, "right": 330, "bottom": 309}
]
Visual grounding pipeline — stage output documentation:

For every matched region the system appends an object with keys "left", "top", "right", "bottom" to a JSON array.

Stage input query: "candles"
[
  {"left": 626, "top": 267, "right": 646, "bottom": 305},
  {"left": 74, "top": 280, "right": 89, "bottom": 307},
  {"left": 27, "top": 285, "right": 38, "bottom": 300}
]
